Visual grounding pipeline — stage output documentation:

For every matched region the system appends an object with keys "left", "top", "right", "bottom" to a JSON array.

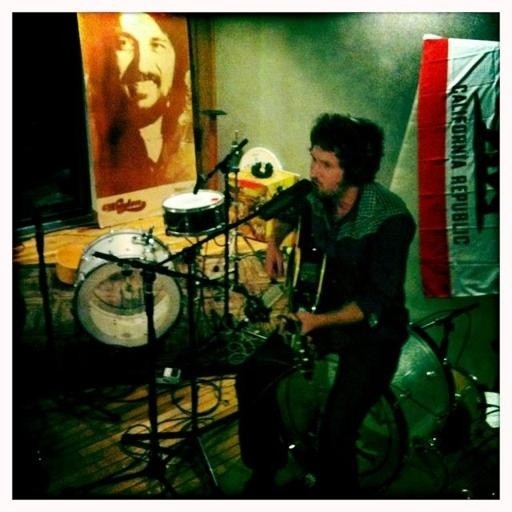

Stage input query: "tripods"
[
  {"left": 192, "top": 149, "right": 269, "bottom": 352},
  {"left": 123, "top": 212, "right": 258, "bottom": 500},
  {"left": 27, "top": 202, "right": 122, "bottom": 422},
  {"left": 58, "top": 250, "right": 232, "bottom": 500}
]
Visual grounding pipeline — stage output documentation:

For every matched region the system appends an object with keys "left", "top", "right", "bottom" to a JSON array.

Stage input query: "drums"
[
  {"left": 277, "top": 322, "right": 456, "bottom": 491},
  {"left": 444, "top": 366, "right": 485, "bottom": 449},
  {"left": 75, "top": 230, "right": 180, "bottom": 347},
  {"left": 163, "top": 193, "right": 228, "bottom": 235}
]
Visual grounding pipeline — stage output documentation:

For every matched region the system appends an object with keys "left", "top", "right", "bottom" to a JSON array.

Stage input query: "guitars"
[{"left": 289, "top": 214, "right": 326, "bottom": 379}]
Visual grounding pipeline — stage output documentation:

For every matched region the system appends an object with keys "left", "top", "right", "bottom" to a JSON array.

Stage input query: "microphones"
[{"left": 259, "top": 179, "right": 313, "bottom": 221}]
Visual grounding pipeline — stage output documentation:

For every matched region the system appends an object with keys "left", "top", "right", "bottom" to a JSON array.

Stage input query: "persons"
[
  {"left": 85, "top": 14, "right": 194, "bottom": 198},
  {"left": 233, "top": 112, "right": 415, "bottom": 497}
]
